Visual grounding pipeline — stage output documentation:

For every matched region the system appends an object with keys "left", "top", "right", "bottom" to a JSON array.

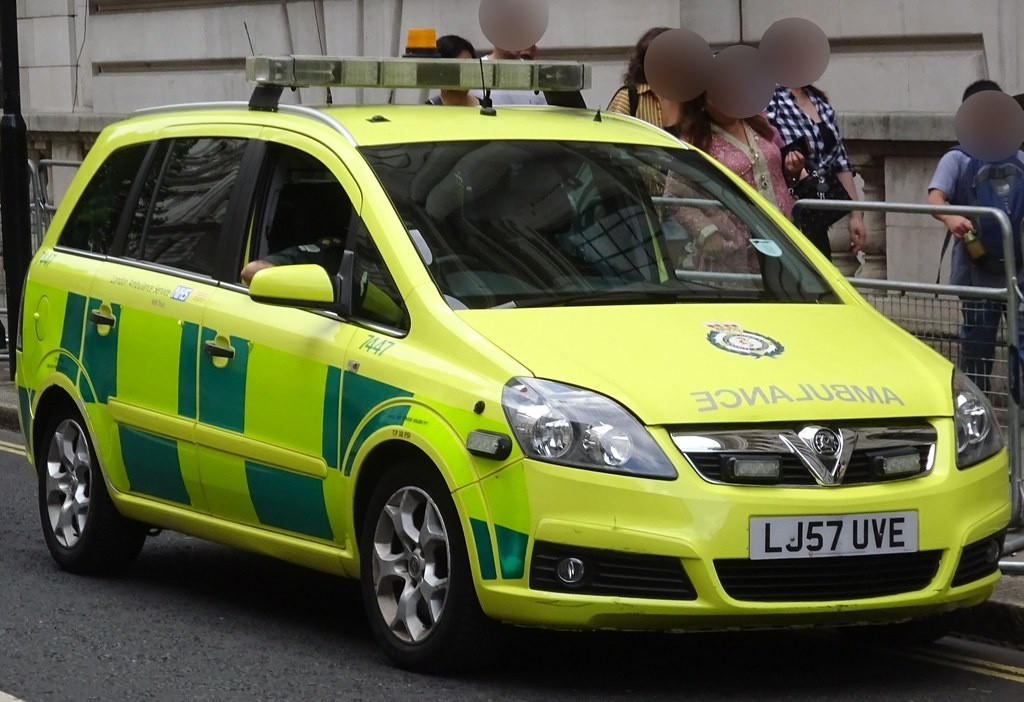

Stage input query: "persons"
[
  {"left": 928, "top": 79, "right": 1024, "bottom": 405},
  {"left": 242, "top": 200, "right": 411, "bottom": 328},
  {"left": 424, "top": 27, "right": 867, "bottom": 295}
]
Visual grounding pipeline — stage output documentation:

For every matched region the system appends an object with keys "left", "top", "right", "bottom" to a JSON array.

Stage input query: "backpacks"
[{"left": 949, "top": 146, "right": 1024, "bottom": 275}]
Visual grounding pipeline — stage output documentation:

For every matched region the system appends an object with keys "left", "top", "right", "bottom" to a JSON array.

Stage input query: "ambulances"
[{"left": 15, "top": 28, "right": 1017, "bottom": 676}]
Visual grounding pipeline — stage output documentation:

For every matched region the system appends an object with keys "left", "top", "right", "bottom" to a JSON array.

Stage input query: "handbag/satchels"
[{"left": 781, "top": 151, "right": 853, "bottom": 234}]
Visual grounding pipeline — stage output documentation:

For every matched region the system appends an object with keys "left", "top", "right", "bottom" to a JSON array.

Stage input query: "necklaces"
[
  {"left": 735, "top": 123, "right": 759, "bottom": 179},
  {"left": 748, "top": 147, "right": 770, "bottom": 189}
]
